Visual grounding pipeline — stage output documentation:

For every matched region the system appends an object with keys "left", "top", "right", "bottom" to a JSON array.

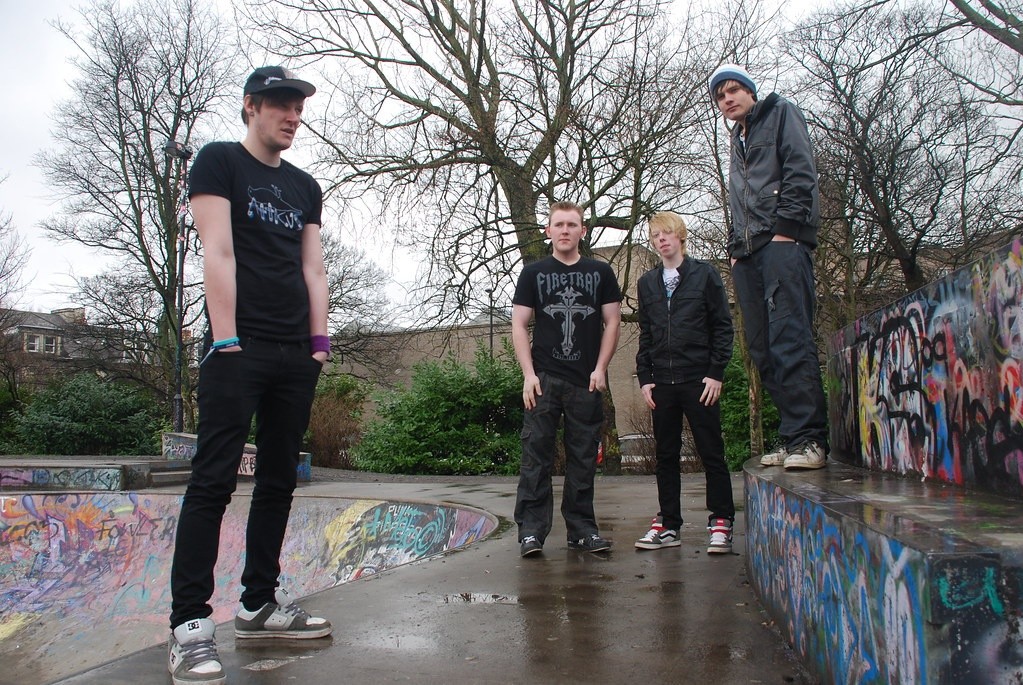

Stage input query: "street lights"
[{"left": 162, "top": 142, "right": 193, "bottom": 433}]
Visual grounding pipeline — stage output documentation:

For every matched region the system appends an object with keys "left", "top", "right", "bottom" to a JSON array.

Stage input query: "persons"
[
  {"left": 164, "top": 65, "right": 337, "bottom": 685},
  {"left": 510, "top": 198, "right": 624, "bottom": 556},
  {"left": 634, "top": 210, "right": 739, "bottom": 554},
  {"left": 705, "top": 61, "right": 833, "bottom": 470}
]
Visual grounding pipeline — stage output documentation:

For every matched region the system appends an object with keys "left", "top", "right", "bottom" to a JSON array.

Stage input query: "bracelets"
[
  {"left": 214, "top": 340, "right": 239, "bottom": 350},
  {"left": 212, "top": 336, "right": 239, "bottom": 347}
]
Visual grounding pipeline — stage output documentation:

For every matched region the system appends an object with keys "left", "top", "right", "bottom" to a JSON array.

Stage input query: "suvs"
[{"left": 595, "top": 432, "right": 698, "bottom": 475}]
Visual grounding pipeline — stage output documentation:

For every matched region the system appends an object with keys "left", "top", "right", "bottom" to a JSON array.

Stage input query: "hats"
[
  {"left": 709, "top": 63, "right": 758, "bottom": 99},
  {"left": 243, "top": 66, "right": 315, "bottom": 98}
]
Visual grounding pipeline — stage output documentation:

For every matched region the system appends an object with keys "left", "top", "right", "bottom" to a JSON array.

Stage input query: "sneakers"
[
  {"left": 567, "top": 532, "right": 611, "bottom": 552},
  {"left": 783, "top": 441, "right": 826, "bottom": 469},
  {"left": 168, "top": 618, "right": 228, "bottom": 685},
  {"left": 707, "top": 514, "right": 733, "bottom": 554},
  {"left": 234, "top": 586, "right": 334, "bottom": 638},
  {"left": 635, "top": 516, "right": 683, "bottom": 550},
  {"left": 760, "top": 447, "right": 790, "bottom": 465},
  {"left": 520, "top": 536, "right": 543, "bottom": 557}
]
